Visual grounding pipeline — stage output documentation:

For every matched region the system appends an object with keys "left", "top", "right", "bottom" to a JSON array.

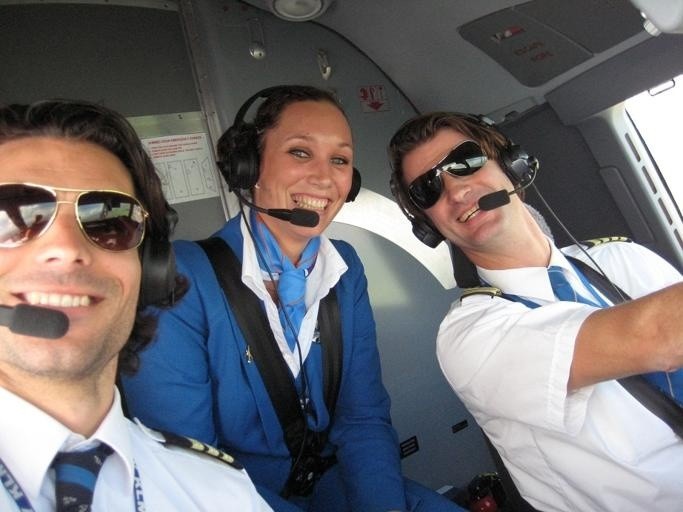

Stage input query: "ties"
[
  {"left": 547, "top": 266, "right": 683, "bottom": 407},
  {"left": 51, "top": 443, "right": 113, "bottom": 512}
]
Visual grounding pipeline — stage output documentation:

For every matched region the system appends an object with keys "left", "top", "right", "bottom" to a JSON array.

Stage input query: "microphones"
[
  {"left": 478, "top": 164, "right": 538, "bottom": 211},
  {"left": 1, "top": 304, "right": 70, "bottom": 339},
  {"left": 213, "top": 163, "right": 320, "bottom": 228}
]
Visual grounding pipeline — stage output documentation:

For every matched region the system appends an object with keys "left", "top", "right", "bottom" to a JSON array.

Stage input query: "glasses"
[
  {"left": 0, "top": 182, "right": 150, "bottom": 252},
  {"left": 407, "top": 139, "right": 488, "bottom": 209}
]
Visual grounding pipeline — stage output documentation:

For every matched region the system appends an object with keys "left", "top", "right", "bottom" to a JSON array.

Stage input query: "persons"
[
  {"left": 388, "top": 111, "right": 683, "bottom": 512},
  {"left": 122, "top": 84, "right": 466, "bottom": 512},
  {"left": 0, "top": 99, "right": 276, "bottom": 512}
]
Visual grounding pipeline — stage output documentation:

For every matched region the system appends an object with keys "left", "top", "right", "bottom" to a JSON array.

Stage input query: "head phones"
[
  {"left": 137, "top": 206, "right": 179, "bottom": 313},
  {"left": 390, "top": 109, "right": 536, "bottom": 248},
  {"left": 216, "top": 85, "right": 360, "bottom": 202}
]
